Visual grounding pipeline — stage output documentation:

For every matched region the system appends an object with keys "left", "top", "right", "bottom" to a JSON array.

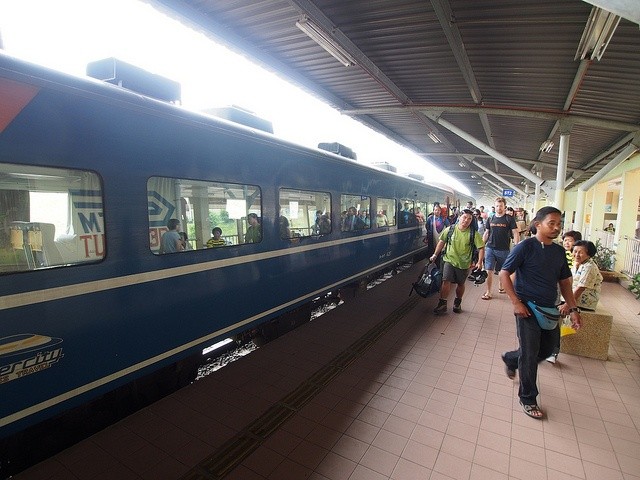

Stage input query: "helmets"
[{"left": 609, "top": 223, "right": 613, "bottom": 227}]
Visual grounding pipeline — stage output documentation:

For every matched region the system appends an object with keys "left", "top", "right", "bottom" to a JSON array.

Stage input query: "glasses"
[{"left": 474, "top": 214, "right": 479, "bottom": 215}]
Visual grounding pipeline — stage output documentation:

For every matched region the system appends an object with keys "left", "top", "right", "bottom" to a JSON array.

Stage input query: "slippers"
[
  {"left": 499, "top": 289, "right": 506, "bottom": 293},
  {"left": 482, "top": 293, "right": 492, "bottom": 300}
]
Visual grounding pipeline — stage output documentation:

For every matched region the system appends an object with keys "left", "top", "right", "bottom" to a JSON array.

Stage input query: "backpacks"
[{"left": 409, "top": 258, "right": 442, "bottom": 298}]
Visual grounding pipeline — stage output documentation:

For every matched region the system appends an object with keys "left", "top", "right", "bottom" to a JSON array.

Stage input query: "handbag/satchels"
[
  {"left": 527, "top": 297, "right": 560, "bottom": 330},
  {"left": 433, "top": 226, "right": 446, "bottom": 252},
  {"left": 560, "top": 315, "right": 576, "bottom": 337}
]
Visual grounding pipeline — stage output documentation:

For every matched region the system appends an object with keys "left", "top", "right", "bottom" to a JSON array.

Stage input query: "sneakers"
[
  {"left": 453, "top": 297, "right": 461, "bottom": 313},
  {"left": 545, "top": 353, "right": 558, "bottom": 364},
  {"left": 433, "top": 298, "right": 447, "bottom": 313}
]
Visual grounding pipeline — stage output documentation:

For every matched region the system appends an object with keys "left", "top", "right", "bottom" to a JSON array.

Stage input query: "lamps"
[
  {"left": 294, "top": 14, "right": 357, "bottom": 68},
  {"left": 538, "top": 139, "right": 554, "bottom": 153},
  {"left": 572, "top": 6, "right": 622, "bottom": 62},
  {"left": 425, "top": 131, "right": 441, "bottom": 144}
]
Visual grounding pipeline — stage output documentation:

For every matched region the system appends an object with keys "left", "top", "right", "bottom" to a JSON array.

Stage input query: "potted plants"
[{"left": 591, "top": 237, "right": 618, "bottom": 280}]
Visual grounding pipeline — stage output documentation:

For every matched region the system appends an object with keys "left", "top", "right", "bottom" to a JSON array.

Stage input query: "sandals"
[
  {"left": 468, "top": 267, "right": 481, "bottom": 281},
  {"left": 519, "top": 400, "right": 544, "bottom": 419},
  {"left": 502, "top": 352, "right": 515, "bottom": 379},
  {"left": 475, "top": 271, "right": 487, "bottom": 284}
]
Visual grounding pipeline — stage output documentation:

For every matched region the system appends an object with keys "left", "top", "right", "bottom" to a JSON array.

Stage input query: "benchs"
[
  {"left": 10, "top": 221, "right": 65, "bottom": 270},
  {"left": 560, "top": 301, "right": 613, "bottom": 361}
]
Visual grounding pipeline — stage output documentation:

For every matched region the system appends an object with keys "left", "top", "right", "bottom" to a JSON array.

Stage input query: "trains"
[{"left": 0, "top": 53, "right": 476, "bottom": 440}]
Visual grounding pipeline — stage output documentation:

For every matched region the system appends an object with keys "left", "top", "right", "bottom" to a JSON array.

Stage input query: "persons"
[
  {"left": 314, "top": 210, "right": 331, "bottom": 235},
  {"left": 377, "top": 210, "right": 389, "bottom": 227},
  {"left": 466, "top": 201, "right": 474, "bottom": 211},
  {"left": 206, "top": 227, "right": 226, "bottom": 248},
  {"left": 545, "top": 240, "right": 604, "bottom": 365},
  {"left": 429, "top": 209, "right": 486, "bottom": 313},
  {"left": 160, "top": 219, "right": 181, "bottom": 254},
  {"left": 489, "top": 207, "right": 496, "bottom": 217},
  {"left": 179, "top": 232, "right": 193, "bottom": 250},
  {"left": 399, "top": 202, "right": 426, "bottom": 224},
  {"left": 514, "top": 207, "right": 532, "bottom": 242},
  {"left": 425, "top": 204, "right": 445, "bottom": 265},
  {"left": 507, "top": 207, "right": 514, "bottom": 216},
  {"left": 279, "top": 204, "right": 290, "bottom": 239},
  {"left": 341, "top": 206, "right": 370, "bottom": 231},
  {"left": 562, "top": 232, "right": 581, "bottom": 273},
  {"left": 245, "top": 213, "right": 262, "bottom": 244},
  {"left": 498, "top": 207, "right": 583, "bottom": 419},
  {"left": 481, "top": 197, "right": 519, "bottom": 300},
  {"left": 480, "top": 206, "right": 488, "bottom": 223},
  {"left": 604, "top": 223, "right": 615, "bottom": 232},
  {"left": 473, "top": 209, "right": 484, "bottom": 237}
]
